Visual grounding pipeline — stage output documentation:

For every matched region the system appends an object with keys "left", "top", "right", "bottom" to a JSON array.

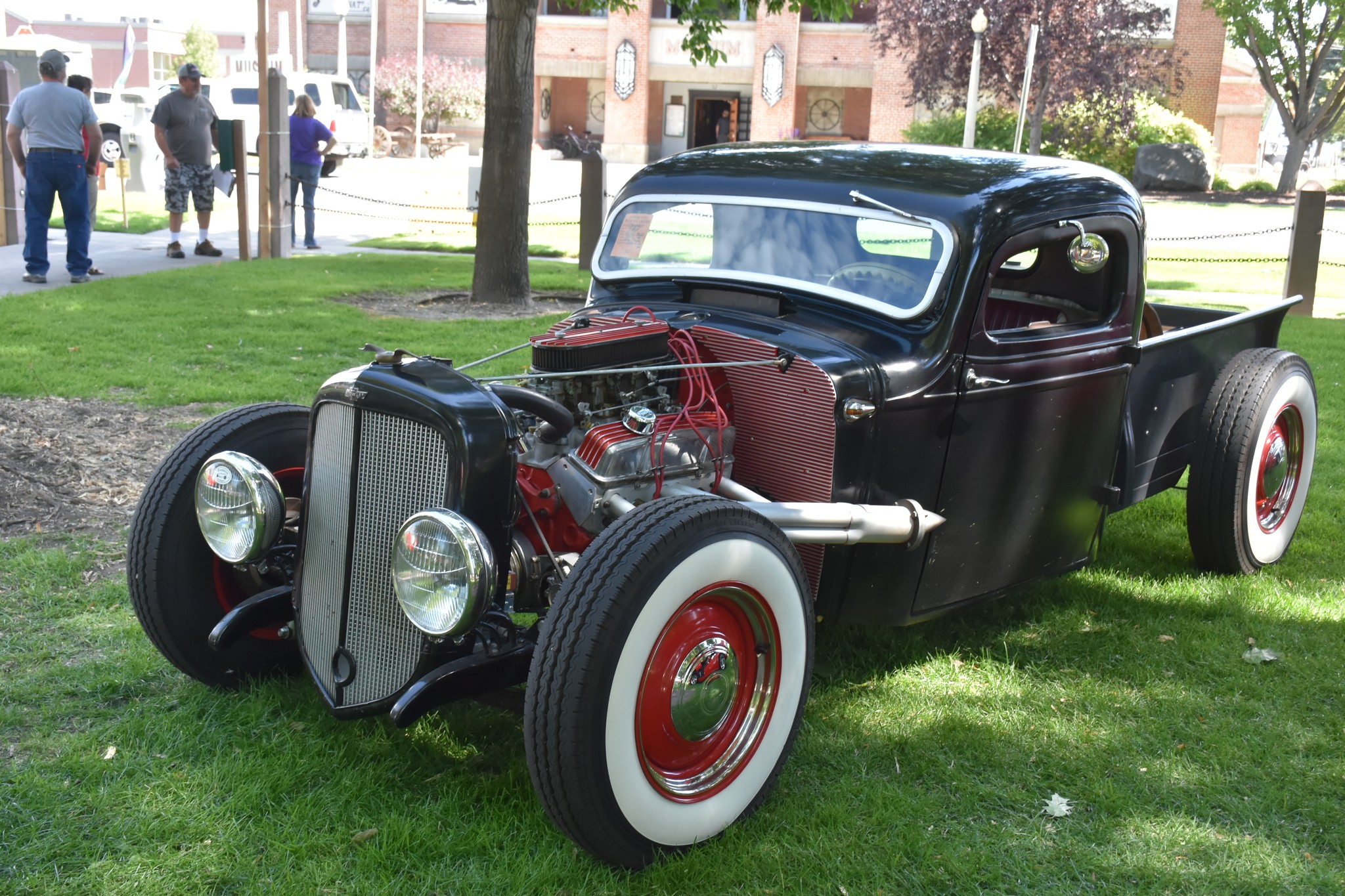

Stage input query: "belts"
[{"left": 29, "top": 147, "right": 83, "bottom": 155}]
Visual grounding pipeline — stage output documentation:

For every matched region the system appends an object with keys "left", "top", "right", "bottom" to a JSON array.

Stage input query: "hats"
[
  {"left": 39, "top": 49, "right": 70, "bottom": 72},
  {"left": 178, "top": 63, "right": 206, "bottom": 79}
]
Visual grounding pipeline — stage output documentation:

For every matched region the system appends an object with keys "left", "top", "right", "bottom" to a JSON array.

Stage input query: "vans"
[{"left": 92, "top": 55, "right": 371, "bottom": 178}]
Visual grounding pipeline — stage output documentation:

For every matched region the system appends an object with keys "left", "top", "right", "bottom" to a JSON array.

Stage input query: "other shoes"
[
  {"left": 194, "top": 239, "right": 223, "bottom": 257},
  {"left": 22, "top": 273, "right": 46, "bottom": 283},
  {"left": 166, "top": 241, "right": 185, "bottom": 258},
  {"left": 71, "top": 274, "right": 92, "bottom": 282}
]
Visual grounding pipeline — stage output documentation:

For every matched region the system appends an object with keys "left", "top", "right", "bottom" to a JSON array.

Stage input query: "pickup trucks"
[{"left": 122, "top": 140, "right": 1323, "bottom": 875}]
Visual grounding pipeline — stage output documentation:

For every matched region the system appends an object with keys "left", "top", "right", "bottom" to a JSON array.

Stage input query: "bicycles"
[{"left": 561, "top": 123, "right": 602, "bottom": 158}]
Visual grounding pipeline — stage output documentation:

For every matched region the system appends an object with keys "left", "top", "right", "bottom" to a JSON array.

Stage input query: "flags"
[{"left": 110, "top": 24, "right": 137, "bottom": 120}]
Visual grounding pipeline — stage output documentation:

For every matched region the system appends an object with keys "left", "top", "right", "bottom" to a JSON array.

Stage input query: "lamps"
[
  {"left": 762, "top": 42, "right": 785, "bottom": 109},
  {"left": 614, "top": 39, "right": 635, "bottom": 100}
]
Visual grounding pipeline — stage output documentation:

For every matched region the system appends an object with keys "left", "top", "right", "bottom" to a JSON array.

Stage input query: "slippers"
[{"left": 89, "top": 267, "right": 104, "bottom": 274}]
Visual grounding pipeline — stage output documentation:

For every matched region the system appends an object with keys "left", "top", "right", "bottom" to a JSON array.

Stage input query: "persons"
[
  {"left": 7, "top": 49, "right": 95, "bottom": 283},
  {"left": 289, "top": 95, "right": 336, "bottom": 252},
  {"left": 152, "top": 63, "right": 231, "bottom": 258},
  {"left": 65, "top": 72, "right": 104, "bottom": 276}
]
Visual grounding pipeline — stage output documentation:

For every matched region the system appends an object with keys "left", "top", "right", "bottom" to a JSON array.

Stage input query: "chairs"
[{"left": 984, "top": 289, "right": 1084, "bottom": 331}]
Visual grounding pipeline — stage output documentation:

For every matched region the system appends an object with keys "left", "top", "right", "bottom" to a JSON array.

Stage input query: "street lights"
[{"left": 962, "top": 7, "right": 989, "bottom": 149}]
